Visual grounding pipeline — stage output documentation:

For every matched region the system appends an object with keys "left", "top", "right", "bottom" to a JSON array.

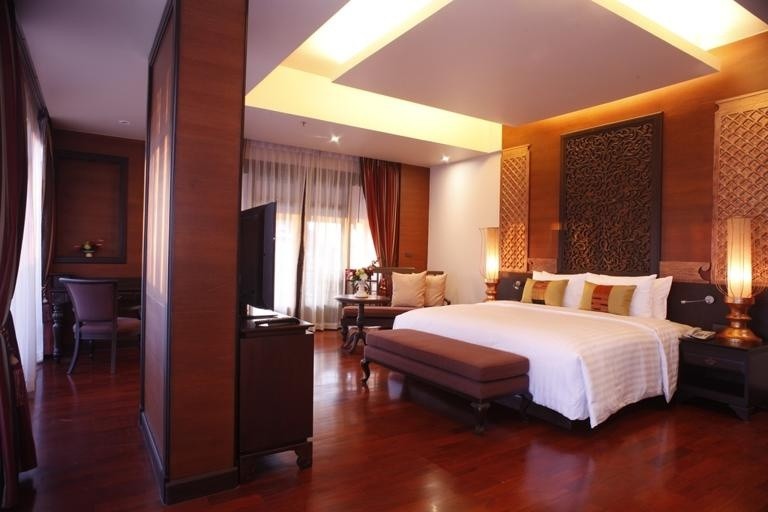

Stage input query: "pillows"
[
  {"left": 410, "top": 271, "right": 447, "bottom": 307},
  {"left": 390, "top": 270, "right": 427, "bottom": 307},
  {"left": 578, "top": 280, "right": 636, "bottom": 317},
  {"left": 541, "top": 268, "right": 548, "bottom": 274},
  {"left": 586, "top": 279, "right": 674, "bottom": 319},
  {"left": 520, "top": 277, "right": 569, "bottom": 307},
  {"left": 586, "top": 271, "right": 658, "bottom": 279},
  {"left": 530, "top": 270, "right": 586, "bottom": 304}
]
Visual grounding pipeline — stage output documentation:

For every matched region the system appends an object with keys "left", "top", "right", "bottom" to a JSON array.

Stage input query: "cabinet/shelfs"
[{"left": 237, "top": 304, "right": 315, "bottom": 486}]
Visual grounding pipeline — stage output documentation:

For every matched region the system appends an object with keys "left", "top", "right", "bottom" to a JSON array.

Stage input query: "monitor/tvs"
[{"left": 237, "top": 201, "right": 279, "bottom": 319}]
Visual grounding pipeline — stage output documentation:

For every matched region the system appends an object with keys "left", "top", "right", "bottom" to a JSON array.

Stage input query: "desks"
[
  {"left": 332, "top": 294, "right": 389, "bottom": 353},
  {"left": 47, "top": 280, "right": 142, "bottom": 362}
]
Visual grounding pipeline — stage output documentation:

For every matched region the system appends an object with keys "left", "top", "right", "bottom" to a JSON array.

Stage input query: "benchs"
[
  {"left": 359, "top": 328, "right": 533, "bottom": 435},
  {"left": 339, "top": 266, "right": 451, "bottom": 347}
]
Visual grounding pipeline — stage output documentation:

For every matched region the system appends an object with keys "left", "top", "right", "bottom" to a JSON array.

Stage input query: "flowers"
[{"left": 346, "top": 260, "right": 377, "bottom": 285}]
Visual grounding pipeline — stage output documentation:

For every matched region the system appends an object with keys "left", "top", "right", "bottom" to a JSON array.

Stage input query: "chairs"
[{"left": 58, "top": 277, "right": 140, "bottom": 376}]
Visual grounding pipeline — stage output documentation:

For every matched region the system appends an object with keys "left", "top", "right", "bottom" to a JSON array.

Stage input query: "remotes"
[{"left": 255, "top": 317, "right": 300, "bottom": 328}]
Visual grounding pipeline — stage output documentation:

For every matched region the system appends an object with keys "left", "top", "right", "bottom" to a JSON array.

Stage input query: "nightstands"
[{"left": 676, "top": 334, "right": 767, "bottom": 422}]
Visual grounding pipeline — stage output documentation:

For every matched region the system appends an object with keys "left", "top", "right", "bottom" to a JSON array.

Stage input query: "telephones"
[{"left": 688, "top": 327, "right": 716, "bottom": 339}]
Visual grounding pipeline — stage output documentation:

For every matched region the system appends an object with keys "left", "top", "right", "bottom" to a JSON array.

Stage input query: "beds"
[{"left": 391, "top": 299, "right": 704, "bottom": 434}]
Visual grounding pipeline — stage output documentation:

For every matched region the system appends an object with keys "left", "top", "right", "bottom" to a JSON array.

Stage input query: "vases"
[{"left": 353, "top": 281, "right": 368, "bottom": 298}]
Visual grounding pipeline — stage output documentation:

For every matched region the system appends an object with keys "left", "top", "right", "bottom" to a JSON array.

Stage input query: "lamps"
[
  {"left": 713, "top": 216, "right": 763, "bottom": 343},
  {"left": 477, "top": 227, "right": 500, "bottom": 302}
]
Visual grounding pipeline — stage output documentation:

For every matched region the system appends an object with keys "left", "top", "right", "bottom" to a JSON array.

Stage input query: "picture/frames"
[{"left": 52, "top": 149, "right": 128, "bottom": 266}]
